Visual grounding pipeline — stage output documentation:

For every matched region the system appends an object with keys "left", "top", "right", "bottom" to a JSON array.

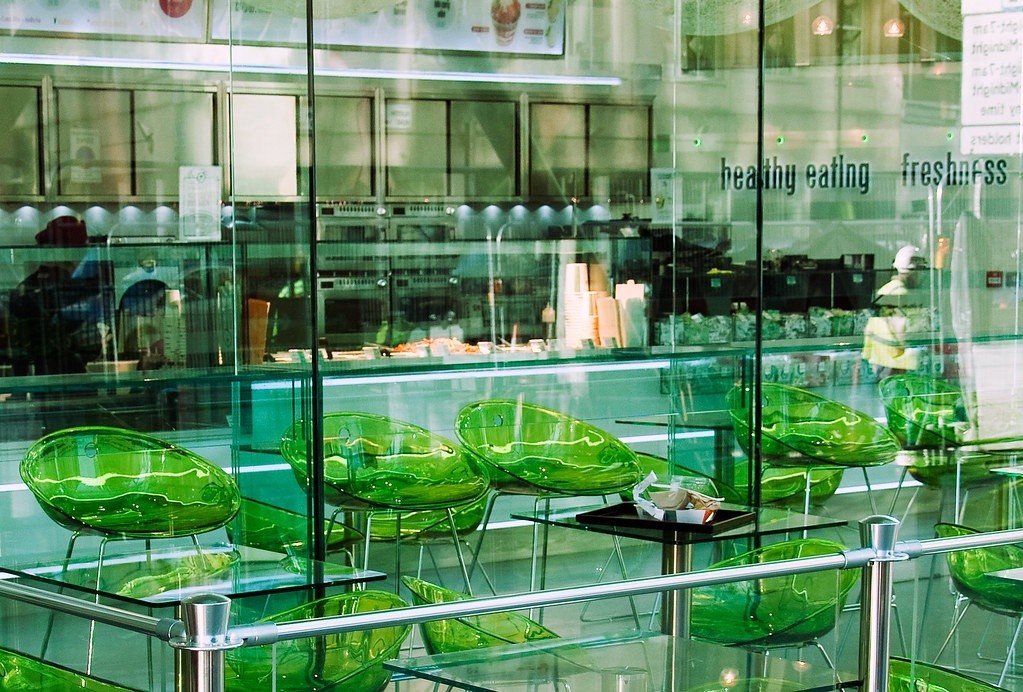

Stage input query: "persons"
[
  {"left": 860, "top": 244, "right": 932, "bottom": 382},
  {"left": 8, "top": 215, "right": 102, "bottom": 458}
]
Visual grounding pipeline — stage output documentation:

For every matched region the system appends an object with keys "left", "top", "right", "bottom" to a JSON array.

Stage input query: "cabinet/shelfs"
[
  {"left": 239, "top": 331, "right": 1023, "bottom": 525},
  {"left": 0, "top": 62, "right": 657, "bottom": 204}
]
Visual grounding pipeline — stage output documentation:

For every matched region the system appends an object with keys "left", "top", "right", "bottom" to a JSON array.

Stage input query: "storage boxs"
[{"left": 655, "top": 260, "right": 877, "bottom": 314}]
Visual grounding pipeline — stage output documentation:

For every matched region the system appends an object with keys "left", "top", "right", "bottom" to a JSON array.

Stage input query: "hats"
[{"left": 34, "top": 217, "right": 89, "bottom": 245}]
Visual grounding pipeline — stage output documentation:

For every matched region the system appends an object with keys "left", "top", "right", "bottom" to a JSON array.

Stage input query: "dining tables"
[
  {"left": 0, "top": 542, "right": 387, "bottom": 692},
  {"left": 384, "top": 632, "right": 865, "bottom": 692},
  {"left": 510, "top": 495, "right": 847, "bottom": 640}
]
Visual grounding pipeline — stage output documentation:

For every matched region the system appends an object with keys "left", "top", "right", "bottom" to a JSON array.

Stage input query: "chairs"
[
  {"left": 224, "top": 590, "right": 413, "bottom": 692},
  {"left": 360, "top": 491, "right": 496, "bottom": 597},
  {"left": 455, "top": 399, "right": 642, "bottom": 631},
  {"left": 877, "top": 375, "right": 969, "bottom": 516},
  {"left": 402, "top": 576, "right": 600, "bottom": 692},
  {"left": 935, "top": 522, "right": 1023, "bottom": 688},
  {"left": 726, "top": 380, "right": 903, "bottom": 514},
  {"left": 692, "top": 539, "right": 860, "bottom": 692},
  {"left": 280, "top": 411, "right": 490, "bottom": 596},
  {"left": 621, "top": 451, "right": 741, "bottom": 503},
  {"left": 226, "top": 495, "right": 363, "bottom": 589},
  {"left": 19, "top": 426, "right": 240, "bottom": 676},
  {"left": 722, "top": 456, "right": 847, "bottom": 539}
]
